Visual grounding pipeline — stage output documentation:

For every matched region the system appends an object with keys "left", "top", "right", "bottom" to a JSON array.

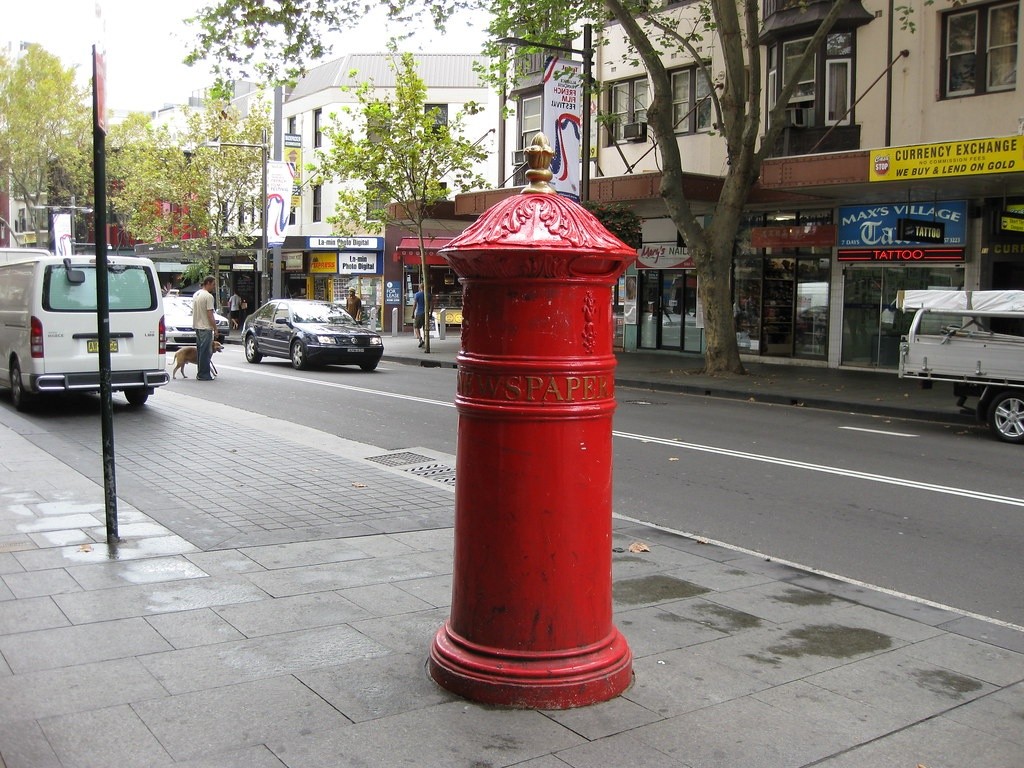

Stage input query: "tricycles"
[{"left": 896, "top": 303, "right": 1024, "bottom": 445}]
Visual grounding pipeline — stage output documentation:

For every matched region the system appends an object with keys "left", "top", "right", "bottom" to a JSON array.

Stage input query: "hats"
[{"left": 348, "top": 288, "right": 355, "bottom": 292}]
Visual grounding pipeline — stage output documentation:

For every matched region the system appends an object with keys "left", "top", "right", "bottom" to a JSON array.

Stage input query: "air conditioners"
[
  {"left": 511, "top": 149, "right": 526, "bottom": 165},
  {"left": 622, "top": 121, "right": 648, "bottom": 143},
  {"left": 784, "top": 106, "right": 807, "bottom": 127}
]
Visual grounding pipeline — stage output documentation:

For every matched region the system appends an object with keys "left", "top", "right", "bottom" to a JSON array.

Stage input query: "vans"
[
  {"left": 0, "top": 249, "right": 53, "bottom": 272},
  {"left": 0, "top": 255, "right": 171, "bottom": 407}
]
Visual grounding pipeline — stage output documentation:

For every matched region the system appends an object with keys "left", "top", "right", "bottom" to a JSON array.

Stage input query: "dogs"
[{"left": 167, "top": 340, "right": 225, "bottom": 380}]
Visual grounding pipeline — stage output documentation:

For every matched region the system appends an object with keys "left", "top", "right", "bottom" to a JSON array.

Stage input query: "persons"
[
  {"left": 347, "top": 288, "right": 361, "bottom": 323},
  {"left": 229, "top": 290, "right": 242, "bottom": 330},
  {"left": 411, "top": 284, "right": 434, "bottom": 347},
  {"left": 193, "top": 277, "right": 218, "bottom": 380}
]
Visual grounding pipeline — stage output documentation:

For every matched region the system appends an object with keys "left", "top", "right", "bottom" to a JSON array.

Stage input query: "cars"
[
  {"left": 241, "top": 298, "right": 385, "bottom": 372},
  {"left": 797, "top": 305, "right": 828, "bottom": 345},
  {"left": 160, "top": 294, "right": 230, "bottom": 349}
]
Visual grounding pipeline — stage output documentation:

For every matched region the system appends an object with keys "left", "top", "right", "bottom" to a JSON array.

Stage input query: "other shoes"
[
  {"left": 419, "top": 341, "right": 424, "bottom": 347},
  {"left": 198, "top": 376, "right": 214, "bottom": 380}
]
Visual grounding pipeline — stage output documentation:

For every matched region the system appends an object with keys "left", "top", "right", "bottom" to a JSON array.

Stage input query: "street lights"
[{"left": 199, "top": 140, "right": 270, "bottom": 306}]
